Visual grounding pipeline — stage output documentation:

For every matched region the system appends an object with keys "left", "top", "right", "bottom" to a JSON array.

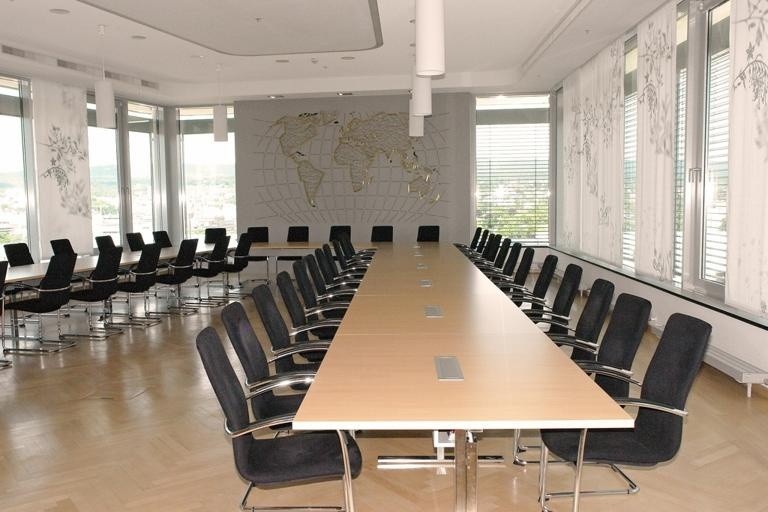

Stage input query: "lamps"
[
  {"left": 413, "top": 0, "right": 447, "bottom": 78},
  {"left": 212, "top": 65, "right": 229, "bottom": 143},
  {"left": 408, "top": 18, "right": 434, "bottom": 117},
  {"left": 94, "top": 23, "right": 118, "bottom": 128},
  {"left": 408, "top": 42, "right": 425, "bottom": 138}
]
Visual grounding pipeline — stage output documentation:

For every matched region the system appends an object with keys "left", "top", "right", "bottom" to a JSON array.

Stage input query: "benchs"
[{"left": 650, "top": 317, "right": 768, "bottom": 398}]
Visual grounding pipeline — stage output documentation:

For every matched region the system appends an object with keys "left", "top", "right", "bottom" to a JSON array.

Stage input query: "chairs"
[
  {"left": 196, "top": 223, "right": 710, "bottom": 511},
  {"left": 0, "top": 227, "right": 199, "bottom": 368}
]
